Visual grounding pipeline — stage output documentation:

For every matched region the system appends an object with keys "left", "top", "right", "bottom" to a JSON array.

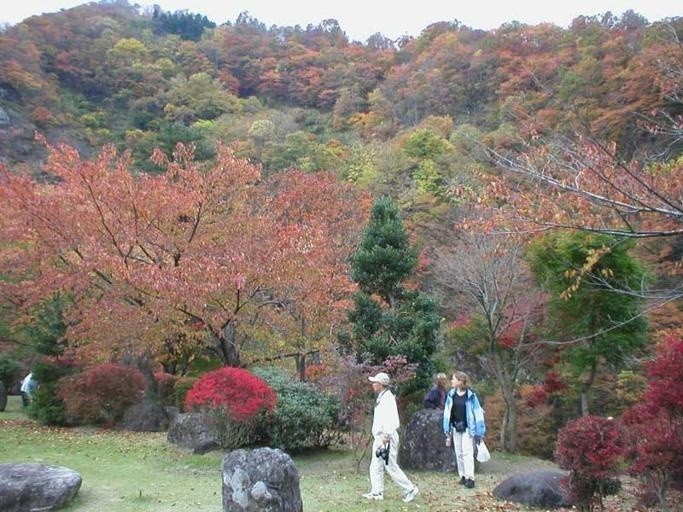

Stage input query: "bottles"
[{"left": 445, "top": 430, "right": 452, "bottom": 447}]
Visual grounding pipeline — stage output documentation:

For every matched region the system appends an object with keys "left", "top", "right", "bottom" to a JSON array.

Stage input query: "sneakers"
[
  {"left": 458, "top": 477, "right": 475, "bottom": 488},
  {"left": 403, "top": 487, "right": 417, "bottom": 501},
  {"left": 363, "top": 492, "right": 383, "bottom": 501}
]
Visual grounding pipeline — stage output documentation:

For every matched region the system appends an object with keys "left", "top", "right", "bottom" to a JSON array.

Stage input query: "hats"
[{"left": 368, "top": 372, "right": 390, "bottom": 386}]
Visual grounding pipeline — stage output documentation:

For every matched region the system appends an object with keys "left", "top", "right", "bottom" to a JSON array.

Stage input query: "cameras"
[{"left": 377, "top": 449, "right": 386, "bottom": 459}]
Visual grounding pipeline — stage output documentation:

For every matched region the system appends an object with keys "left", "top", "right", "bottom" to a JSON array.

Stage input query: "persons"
[
  {"left": 444, "top": 370, "right": 486, "bottom": 489},
  {"left": 422, "top": 372, "right": 449, "bottom": 409},
  {"left": 20, "top": 372, "right": 41, "bottom": 407},
  {"left": 362, "top": 372, "right": 419, "bottom": 504}
]
